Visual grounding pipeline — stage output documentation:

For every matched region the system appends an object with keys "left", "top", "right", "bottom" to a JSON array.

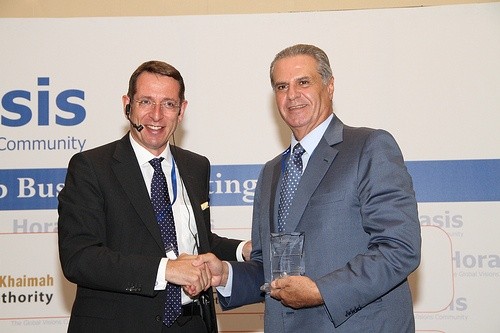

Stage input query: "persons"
[
  {"left": 182, "top": 44, "right": 422, "bottom": 333},
  {"left": 57, "top": 60, "right": 253, "bottom": 333}
]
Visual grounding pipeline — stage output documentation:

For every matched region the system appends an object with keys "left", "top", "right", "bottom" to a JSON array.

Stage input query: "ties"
[
  {"left": 278, "top": 143, "right": 306, "bottom": 233},
  {"left": 148, "top": 157, "right": 182, "bottom": 327}
]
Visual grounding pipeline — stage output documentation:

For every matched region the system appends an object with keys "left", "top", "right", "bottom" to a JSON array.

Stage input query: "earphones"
[{"left": 125, "top": 103, "right": 130, "bottom": 115}]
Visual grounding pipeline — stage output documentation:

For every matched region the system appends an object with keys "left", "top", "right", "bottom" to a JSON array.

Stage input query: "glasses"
[{"left": 129, "top": 97, "right": 181, "bottom": 110}]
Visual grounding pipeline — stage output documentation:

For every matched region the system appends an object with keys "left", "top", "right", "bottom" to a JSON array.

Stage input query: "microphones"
[{"left": 126, "top": 114, "right": 144, "bottom": 132}]
